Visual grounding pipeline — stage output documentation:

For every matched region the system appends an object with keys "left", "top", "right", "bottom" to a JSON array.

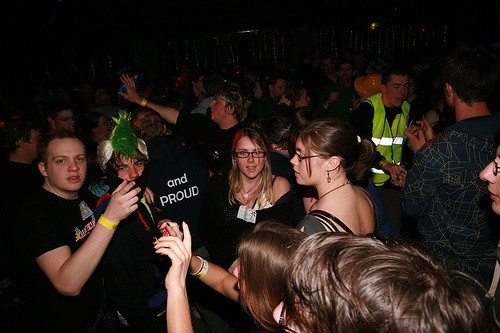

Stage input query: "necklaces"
[
  {"left": 306, "top": 180, "right": 351, "bottom": 214},
  {"left": 241, "top": 177, "right": 261, "bottom": 199}
]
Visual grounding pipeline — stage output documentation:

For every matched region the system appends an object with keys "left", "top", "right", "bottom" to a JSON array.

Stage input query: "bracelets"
[
  {"left": 188, "top": 256, "right": 208, "bottom": 279},
  {"left": 98, "top": 213, "right": 119, "bottom": 231},
  {"left": 140, "top": 96, "right": 148, "bottom": 106},
  {"left": 380, "top": 160, "right": 390, "bottom": 169}
]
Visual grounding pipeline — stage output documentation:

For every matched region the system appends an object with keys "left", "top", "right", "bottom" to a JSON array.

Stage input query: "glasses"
[
  {"left": 295, "top": 151, "right": 324, "bottom": 161},
  {"left": 56, "top": 116, "right": 75, "bottom": 122},
  {"left": 488, "top": 154, "right": 500, "bottom": 176},
  {"left": 234, "top": 151, "right": 267, "bottom": 158}
]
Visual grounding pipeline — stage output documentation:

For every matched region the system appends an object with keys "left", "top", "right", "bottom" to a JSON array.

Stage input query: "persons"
[{"left": 0, "top": 45, "right": 500, "bottom": 333}]
